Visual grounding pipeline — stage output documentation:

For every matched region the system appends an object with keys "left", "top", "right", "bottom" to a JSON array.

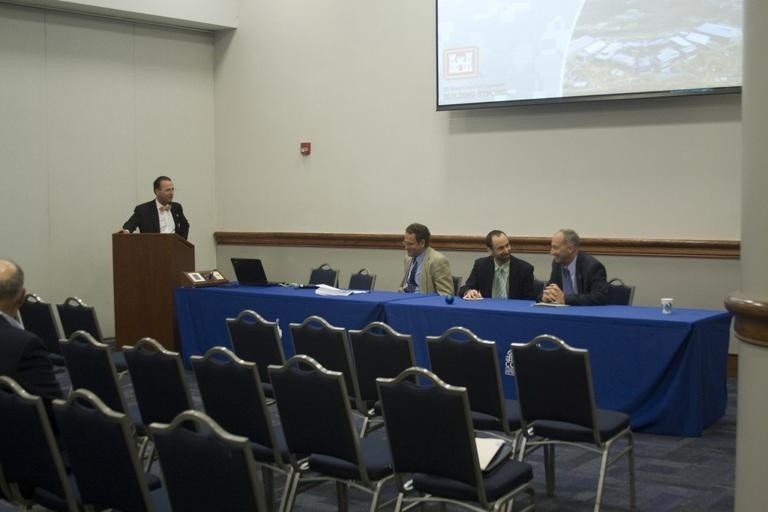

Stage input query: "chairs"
[
  {"left": 452, "top": 276, "right": 461, "bottom": 295},
  {"left": 348, "top": 267, "right": 376, "bottom": 290},
  {"left": 533, "top": 280, "right": 544, "bottom": 299},
  {"left": 309, "top": 263, "right": 340, "bottom": 288},
  {"left": 607, "top": 278, "right": 635, "bottom": 306}
]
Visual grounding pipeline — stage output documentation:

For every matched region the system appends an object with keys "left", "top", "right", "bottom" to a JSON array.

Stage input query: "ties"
[
  {"left": 410, "top": 262, "right": 418, "bottom": 286},
  {"left": 496, "top": 268, "right": 507, "bottom": 298},
  {"left": 564, "top": 269, "right": 573, "bottom": 295}
]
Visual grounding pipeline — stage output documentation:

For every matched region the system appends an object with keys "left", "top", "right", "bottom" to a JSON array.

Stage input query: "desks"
[
  {"left": 172, "top": 281, "right": 438, "bottom": 373},
  {"left": 384, "top": 294, "right": 734, "bottom": 439}
]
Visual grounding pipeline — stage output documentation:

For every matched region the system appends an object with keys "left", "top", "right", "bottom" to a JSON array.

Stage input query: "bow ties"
[{"left": 160, "top": 204, "right": 169, "bottom": 211}]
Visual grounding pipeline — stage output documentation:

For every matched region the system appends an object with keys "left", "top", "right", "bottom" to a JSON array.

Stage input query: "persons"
[
  {"left": 396, "top": 223, "right": 455, "bottom": 297},
  {"left": 457, "top": 229, "right": 536, "bottom": 300},
  {"left": 538, "top": 228, "right": 609, "bottom": 305},
  {"left": 122, "top": 175, "right": 190, "bottom": 240},
  {"left": 1, "top": 258, "right": 66, "bottom": 399}
]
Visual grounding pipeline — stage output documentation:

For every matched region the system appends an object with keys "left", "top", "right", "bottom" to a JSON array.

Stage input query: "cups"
[{"left": 660, "top": 298, "right": 674, "bottom": 315}]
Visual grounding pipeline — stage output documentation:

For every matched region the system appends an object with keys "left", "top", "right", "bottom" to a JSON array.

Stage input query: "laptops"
[{"left": 231, "top": 258, "right": 285, "bottom": 286}]
